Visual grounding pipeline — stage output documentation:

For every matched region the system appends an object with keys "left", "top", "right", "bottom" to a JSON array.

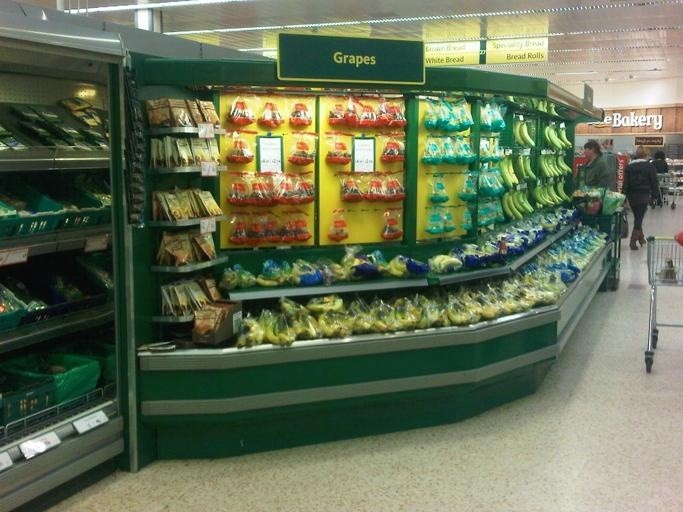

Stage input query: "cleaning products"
[
  {"left": 581, "top": 212, "right": 623, "bottom": 292},
  {"left": 640, "top": 235, "right": 683, "bottom": 372},
  {"left": 656, "top": 173, "right": 679, "bottom": 210}
]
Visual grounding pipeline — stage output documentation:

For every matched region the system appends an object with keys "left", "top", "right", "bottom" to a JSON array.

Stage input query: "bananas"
[{"left": 220, "top": 93, "right": 610, "bottom": 350}]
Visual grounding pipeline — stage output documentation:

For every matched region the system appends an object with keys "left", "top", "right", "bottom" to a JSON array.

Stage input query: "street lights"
[{"left": 613, "top": 211, "right": 628, "bottom": 239}]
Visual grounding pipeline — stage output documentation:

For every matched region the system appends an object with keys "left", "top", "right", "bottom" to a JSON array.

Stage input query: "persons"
[
  {"left": 584, "top": 141, "right": 610, "bottom": 190},
  {"left": 621, "top": 145, "right": 659, "bottom": 250},
  {"left": 652, "top": 151, "right": 668, "bottom": 206}
]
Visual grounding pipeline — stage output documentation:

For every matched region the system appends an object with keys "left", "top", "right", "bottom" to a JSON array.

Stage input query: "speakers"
[
  {"left": 639, "top": 229, "right": 646, "bottom": 247},
  {"left": 630, "top": 229, "right": 639, "bottom": 250}
]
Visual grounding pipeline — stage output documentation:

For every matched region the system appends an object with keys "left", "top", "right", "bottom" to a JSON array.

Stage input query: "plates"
[
  {"left": 123, "top": 51, "right": 620, "bottom": 473},
  {"left": 665, "top": 158, "right": 683, "bottom": 195},
  {"left": 0, "top": 15, "right": 127, "bottom": 511}
]
